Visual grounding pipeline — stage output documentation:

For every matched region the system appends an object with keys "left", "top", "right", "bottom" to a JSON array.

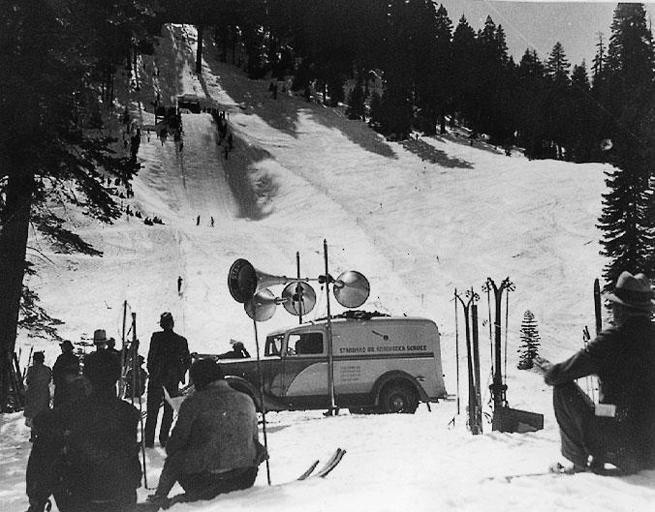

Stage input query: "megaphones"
[
  {"left": 227, "top": 258, "right": 326, "bottom": 304},
  {"left": 244, "top": 288, "right": 288, "bottom": 322},
  {"left": 281, "top": 281, "right": 316, "bottom": 315},
  {"left": 327, "top": 270, "right": 371, "bottom": 308}
]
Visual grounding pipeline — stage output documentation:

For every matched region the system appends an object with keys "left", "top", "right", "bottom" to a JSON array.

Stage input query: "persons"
[
  {"left": 216, "top": 339, "right": 252, "bottom": 359},
  {"left": 146, "top": 356, "right": 259, "bottom": 504},
  {"left": 196, "top": 215, "right": 201, "bottom": 225},
  {"left": 210, "top": 217, "right": 214, "bottom": 227},
  {"left": 144, "top": 311, "right": 191, "bottom": 449},
  {"left": 176, "top": 275, "right": 183, "bottom": 294},
  {"left": 24, "top": 329, "right": 147, "bottom": 512},
  {"left": 543, "top": 271, "right": 655, "bottom": 477}
]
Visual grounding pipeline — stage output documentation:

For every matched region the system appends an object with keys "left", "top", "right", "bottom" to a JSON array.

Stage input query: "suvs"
[{"left": 190, "top": 313, "right": 448, "bottom": 415}]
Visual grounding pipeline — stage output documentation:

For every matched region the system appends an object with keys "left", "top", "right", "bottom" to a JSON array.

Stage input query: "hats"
[{"left": 603, "top": 270, "right": 654, "bottom": 308}]
